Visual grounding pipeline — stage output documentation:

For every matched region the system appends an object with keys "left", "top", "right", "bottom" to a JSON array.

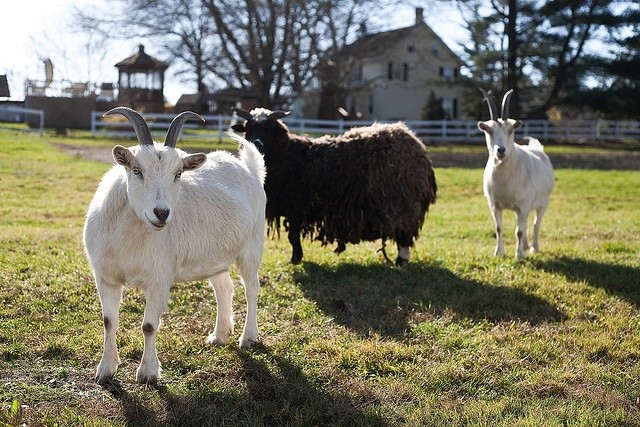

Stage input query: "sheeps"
[
  {"left": 477, "top": 85, "right": 555, "bottom": 260},
  {"left": 232, "top": 107, "right": 438, "bottom": 267},
  {"left": 82, "top": 106, "right": 267, "bottom": 389}
]
